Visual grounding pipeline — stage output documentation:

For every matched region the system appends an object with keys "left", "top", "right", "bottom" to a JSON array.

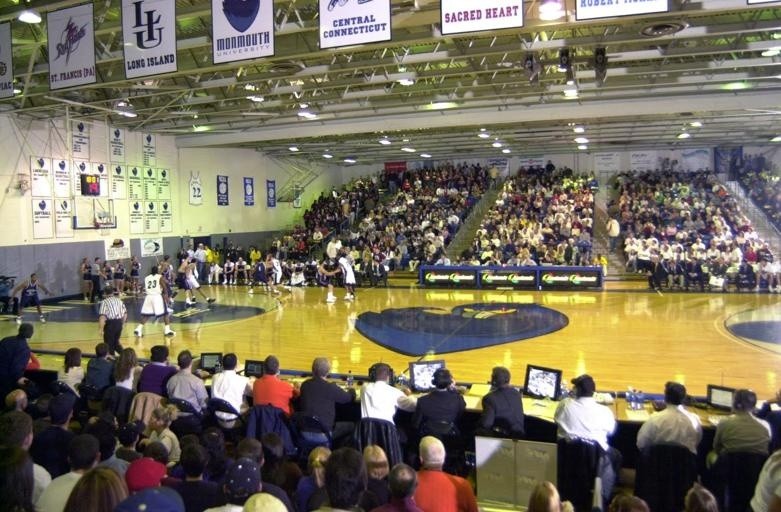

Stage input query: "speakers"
[
  {"left": 524, "top": 55, "right": 533, "bottom": 68},
  {"left": 595, "top": 48, "right": 605, "bottom": 64},
  {"left": 560, "top": 49, "right": 570, "bottom": 68}
]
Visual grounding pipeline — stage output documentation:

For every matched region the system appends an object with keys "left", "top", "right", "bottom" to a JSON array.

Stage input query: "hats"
[
  {"left": 571, "top": 375, "right": 595, "bottom": 392},
  {"left": 49, "top": 393, "right": 261, "bottom": 512}
]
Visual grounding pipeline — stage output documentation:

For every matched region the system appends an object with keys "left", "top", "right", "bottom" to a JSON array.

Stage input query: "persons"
[
  {"left": 80, "top": 256, "right": 141, "bottom": 302},
  {"left": 460, "top": 160, "right": 599, "bottom": 274},
  {"left": 98, "top": 286, "right": 128, "bottom": 355},
  {"left": 477, "top": 367, "right": 524, "bottom": 440},
  {"left": 529, "top": 375, "right": 780, "bottom": 511},
  {"left": 593, "top": 254, "right": 607, "bottom": 277},
  {"left": 606, "top": 216, "right": 620, "bottom": 254},
  {"left": 334, "top": 251, "right": 356, "bottom": 299},
  {"left": 133, "top": 267, "right": 175, "bottom": 337},
  {"left": 1, "top": 343, "right": 477, "bottom": 511},
  {"left": 159, "top": 255, "right": 174, "bottom": 312},
  {"left": 272, "top": 161, "right": 498, "bottom": 289},
  {"left": 9, "top": 273, "right": 54, "bottom": 324},
  {"left": 1, "top": 324, "right": 34, "bottom": 390},
  {"left": 177, "top": 243, "right": 291, "bottom": 294},
  {"left": 615, "top": 152, "right": 780, "bottom": 293},
  {"left": 177, "top": 254, "right": 216, "bottom": 306},
  {"left": 319, "top": 257, "right": 337, "bottom": 302}
]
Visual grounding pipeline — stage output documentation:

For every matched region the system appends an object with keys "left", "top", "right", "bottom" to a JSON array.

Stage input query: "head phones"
[
  {"left": 735, "top": 390, "right": 753, "bottom": 410},
  {"left": 573, "top": 376, "right": 593, "bottom": 395},
  {"left": 492, "top": 367, "right": 509, "bottom": 385},
  {"left": 665, "top": 383, "right": 680, "bottom": 402},
  {"left": 432, "top": 368, "right": 453, "bottom": 385},
  {"left": 369, "top": 364, "right": 393, "bottom": 379}
]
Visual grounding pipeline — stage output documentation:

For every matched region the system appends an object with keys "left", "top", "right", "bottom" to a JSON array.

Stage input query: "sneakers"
[
  {"left": 134, "top": 295, "right": 216, "bottom": 338},
  {"left": 222, "top": 280, "right": 354, "bottom": 303}
]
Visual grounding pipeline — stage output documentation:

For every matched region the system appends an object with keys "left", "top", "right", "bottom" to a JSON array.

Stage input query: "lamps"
[
  {"left": 114, "top": 98, "right": 138, "bottom": 117},
  {"left": 536, "top": 2, "right": 565, "bottom": 21},
  {"left": 677, "top": 120, "right": 703, "bottom": 140},
  {"left": 289, "top": 147, "right": 356, "bottom": 163},
  {"left": 760, "top": 34, "right": 779, "bottom": 58},
  {"left": 379, "top": 138, "right": 432, "bottom": 158},
  {"left": 297, "top": 107, "right": 317, "bottom": 121},
  {"left": 478, "top": 128, "right": 511, "bottom": 154},
  {"left": 14, "top": 80, "right": 21, "bottom": 96},
  {"left": 572, "top": 123, "right": 589, "bottom": 150},
  {"left": 563, "top": 79, "right": 577, "bottom": 99},
  {"left": 243, "top": 82, "right": 264, "bottom": 102},
  {"left": 396, "top": 67, "right": 414, "bottom": 86},
  {"left": 17, "top": 8, "right": 41, "bottom": 26}
]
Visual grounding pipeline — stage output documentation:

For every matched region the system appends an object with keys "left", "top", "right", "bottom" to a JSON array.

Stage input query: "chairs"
[
  {"left": 556, "top": 438, "right": 770, "bottom": 510},
  {"left": 21, "top": 376, "right": 513, "bottom": 480}
]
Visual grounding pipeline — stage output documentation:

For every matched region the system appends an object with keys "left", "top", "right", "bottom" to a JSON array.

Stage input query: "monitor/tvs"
[
  {"left": 408, "top": 360, "right": 445, "bottom": 392},
  {"left": 524, "top": 365, "right": 561, "bottom": 400},
  {"left": 245, "top": 360, "right": 264, "bottom": 378},
  {"left": 707, "top": 384, "right": 736, "bottom": 412},
  {"left": 200, "top": 352, "right": 223, "bottom": 374}
]
